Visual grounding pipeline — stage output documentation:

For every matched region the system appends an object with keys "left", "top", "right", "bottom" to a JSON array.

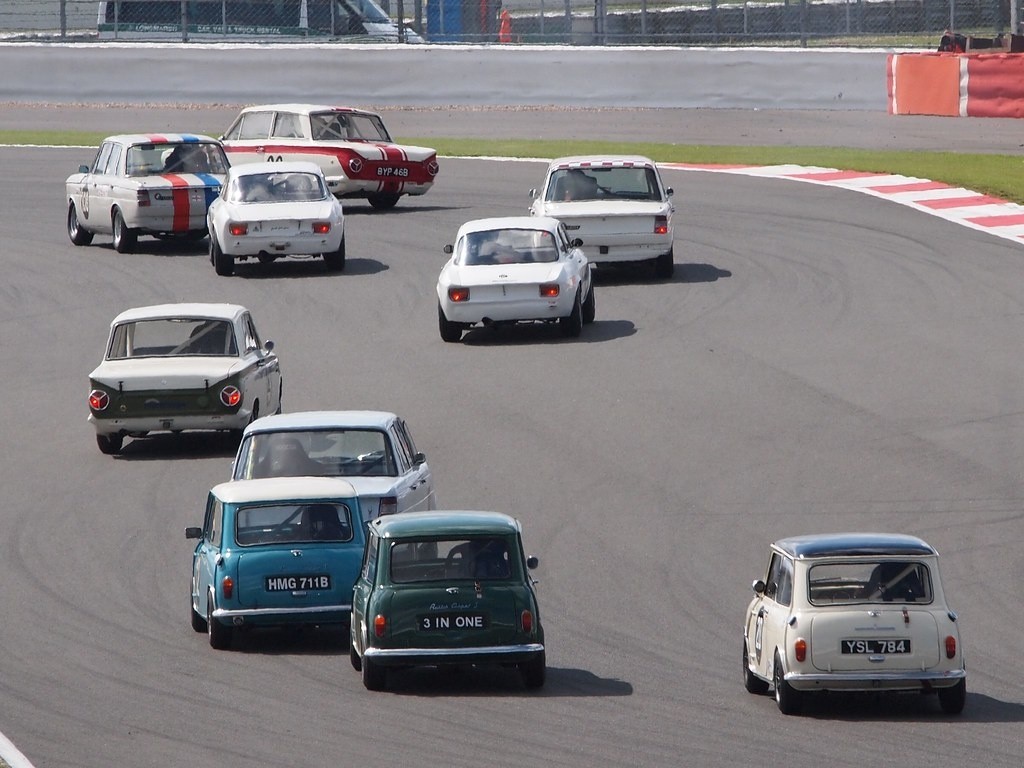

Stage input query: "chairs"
[
  {"left": 254, "top": 438, "right": 326, "bottom": 477},
  {"left": 859, "top": 563, "right": 924, "bottom": 601},
  {"left": 440, "top": 540, "right": 511, "bottom": 579},
  {"left": 293, "top": 504, "right": 350, "bottom": 542},
  {"left": 552, "top": 175, "right": 599, "bottom": 202}
]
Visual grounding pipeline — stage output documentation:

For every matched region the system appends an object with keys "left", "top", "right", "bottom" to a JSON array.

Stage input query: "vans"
[{"left": 98, "top": 0, "right": 423, "bottom": 42}]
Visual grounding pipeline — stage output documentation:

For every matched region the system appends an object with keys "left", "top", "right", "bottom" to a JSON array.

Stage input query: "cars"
[
  {"left": 65, "top": 133, "right": 238, "bottom": 254},
  {"left": 88, "top": 301, "right": 282, "bottom": 456},
  {"left": 530, "top": 154, "right": 678, "bottom": 283},
  {"left": 162, "top": 103, "right": 442, "bottom": 209},
  {"left": 347, "top": 513, "right": 546, "bottom": 689},
  {"left": 437, "top": 216, "right": 598, "bottom": 343},
  {"left": 205, "top": 161, "right": 346, "bottom": 276},
  {"left": 187, "top": 480, "right": 366, "bottom": 649},
  {"left": 740, "top": 532, "right": 967, "bottom": 715},
  {"left": 232, "top": 410, "right": 436, "bottom": 524}
]
